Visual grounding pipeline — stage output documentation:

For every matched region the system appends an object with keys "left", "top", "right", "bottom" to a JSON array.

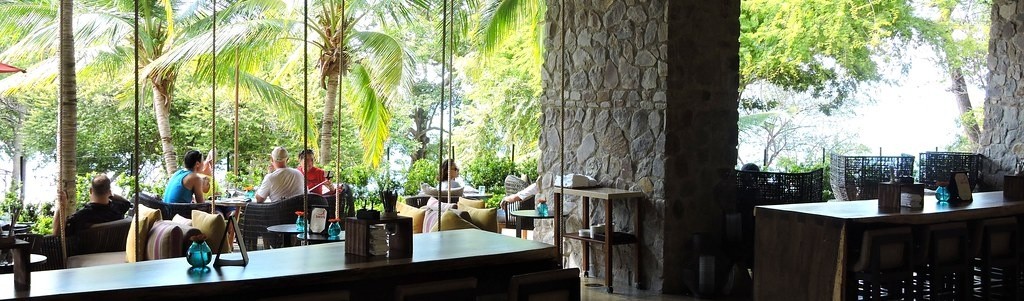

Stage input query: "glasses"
[{"left": 451, "top": 167, "right": 459, "bottom": 172}]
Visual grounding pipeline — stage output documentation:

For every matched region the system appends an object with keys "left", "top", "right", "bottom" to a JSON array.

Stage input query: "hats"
[{"left": 272, "top": 147, "right": 289, "bottom": 162}]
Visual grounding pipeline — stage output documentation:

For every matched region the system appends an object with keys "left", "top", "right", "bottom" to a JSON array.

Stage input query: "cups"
[
  {"left": 590, "top": 226, "right": 601, "bottom": 238},
  {"left": 478, "top": 186, "right": 485, "bottom": 195},
  {"left": 878, "top": 182, "right": 902, "bottom": 212}
]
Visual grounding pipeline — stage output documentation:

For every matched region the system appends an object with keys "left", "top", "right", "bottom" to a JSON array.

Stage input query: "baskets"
[
  {"left": 919, "top": 152, "right": 980, "bottom": 193},
  {"left": 736, "top": 168, "right": 823, "bottom": 209},
  {"left": 830, "top": 152, "right": 915, "bottom": 201}
]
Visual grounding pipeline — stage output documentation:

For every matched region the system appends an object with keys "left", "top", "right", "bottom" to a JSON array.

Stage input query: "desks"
[
  {"left": 267, "top": 224, "right": 310, "bottom": 247},
  {"left": 0, "top": 228, "right": 557, "bottom": 301},
  {"left": 296, "top": 230, "right": 345, "bottom": 245},
  {"left": 0, "top": 254, "right": 47, "bottom": 274},
  {"left": 752, "top": 190, "right": 1024, "bottom": 301},
  {"left": 510, "top": 209, "right": 569, "bottom": 269}
]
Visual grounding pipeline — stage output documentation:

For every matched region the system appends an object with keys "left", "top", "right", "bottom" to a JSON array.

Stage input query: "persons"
[
  {"left": 436, "top": 158, "right": 466, "bottom": 197},
  {"left": 161, "top": 150, "right": 206, "bottom": 204},
  {"left": 254, "top": 144, "right": 309, "bottom": 202},
  {"left": 198, "top": 159, "right": 228, "bottom": 201},
  {"left": 52, "top": 173, "right": 132, "bottom": 238},
  {"left": 293, "top": 148, "right": 336, "bottom": 194},
  {"left": 499, "top": 180, "right": 538, "bottom": 210}
]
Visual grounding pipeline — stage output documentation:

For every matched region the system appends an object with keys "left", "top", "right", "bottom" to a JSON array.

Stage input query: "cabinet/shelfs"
[{"left": 554, "top": 186, "right": 641, "bottom": 294}]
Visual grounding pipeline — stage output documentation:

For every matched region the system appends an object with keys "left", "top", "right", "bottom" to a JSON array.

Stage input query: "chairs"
[
  {"left": 919, "top": 151, "right": 980, "bottom": 190},
  {"left": 830, "top": 151, "right": 915, "bottom": 201},
  {"left": 844, "top": 215, "right": 1024, "bottom": 301},
  {"left": 735, "top": 168, "right": 823, "bottom": 269},
  {"left": 0, "top": 183, "right": 581, "bottom": 301}
]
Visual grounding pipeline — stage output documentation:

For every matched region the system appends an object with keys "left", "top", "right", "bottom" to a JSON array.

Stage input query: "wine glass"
[{"left": 228, "top": 183, "right": 235, "bottom": 199}]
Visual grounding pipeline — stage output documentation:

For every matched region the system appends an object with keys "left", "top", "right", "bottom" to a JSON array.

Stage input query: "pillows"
[
  {"left": 421, "top": 181, "right": 464, "bottom": 196},
  {"left": 172, "top": 214, "right": 194, "bottom": 227},
  {"left": 146, "top": 218, "right": 183, "bottom": 260},
  {"left": 191, "top": 209, "right": 231, "bottom": 254},
  {"left": 429, "top": 208, "right": 482, "bottom": 232},
  {"left": 126, "top": 214, "right": 148, "bottom": 263},
  {"left": 457, "top": 202, "right": 498, "bottom": 233},
  {"left": 427, "top": 196, "right": 458, "bottom": 212},
  {"left": 164, "top": 220, "right": 204, "bottom": 257},
  {"left": 459, "top": 196, "right": 485, "bottom": 209},
  {"left": 126, "top": 203, "right": 163, "bottom": 263},
  {"left": 419, "top": 206, "right": 443, "bottom": 233}
]
